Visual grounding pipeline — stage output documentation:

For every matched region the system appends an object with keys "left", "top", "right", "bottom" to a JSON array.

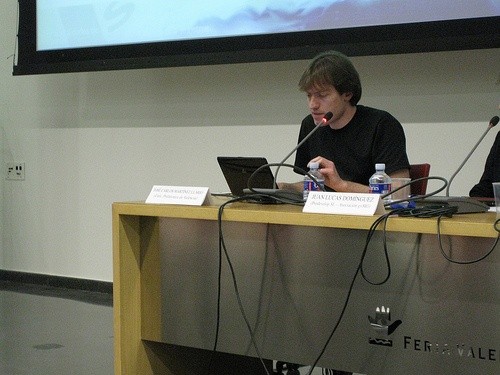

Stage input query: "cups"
[
  {"left": 492, "top": 183, "right": 500, "bottom": 223},
  {"left": 391, "top": 178, "right": 412, "bottom": 204}
]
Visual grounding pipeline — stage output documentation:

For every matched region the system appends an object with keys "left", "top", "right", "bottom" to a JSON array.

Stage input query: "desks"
[{"left": 111, "top": 194, "right": 500, "bottom": 375}]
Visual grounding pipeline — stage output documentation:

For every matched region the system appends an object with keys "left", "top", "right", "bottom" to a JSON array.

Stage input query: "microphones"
[
  {"left": 409, "top": 117, "right": 499, "bottom": 214},
  {"left": 242, "top": 112, "right": 333, "bottom": 203}
]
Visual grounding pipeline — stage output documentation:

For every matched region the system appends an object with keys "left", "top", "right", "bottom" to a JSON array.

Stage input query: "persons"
[
  {"left": 469, "top": 130, "right": 500, "bottom": 206},
  {"left": 275, "top": 52, "right": 412, "bottom": 200}
]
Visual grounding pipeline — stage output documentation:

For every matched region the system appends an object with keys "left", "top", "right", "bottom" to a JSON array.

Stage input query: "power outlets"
[{"left": 4, "top": 165, "right": 26, "bottom": 182}]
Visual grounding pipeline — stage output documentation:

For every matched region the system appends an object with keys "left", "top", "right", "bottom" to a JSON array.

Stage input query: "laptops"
[{"left": 217, "top": 157, "right": 279, "bottom": 196}]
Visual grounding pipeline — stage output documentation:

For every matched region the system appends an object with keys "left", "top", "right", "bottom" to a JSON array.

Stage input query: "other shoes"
[{"left": 322, "top": 368, "right": 353, "bottom": 375}]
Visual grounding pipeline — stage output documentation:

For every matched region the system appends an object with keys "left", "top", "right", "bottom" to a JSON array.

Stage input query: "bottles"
[
  {"left": 303, "top": 162, "right": 325, "bottom": 204},
  {"left": 368, "top": 164, "right": 392, "bottom": 207}
]
